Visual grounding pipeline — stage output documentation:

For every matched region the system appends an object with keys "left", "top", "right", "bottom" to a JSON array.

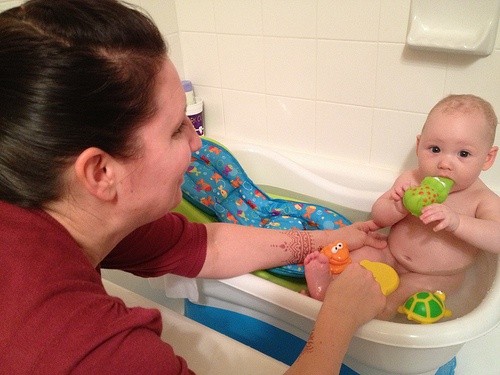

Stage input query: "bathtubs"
[{"left": 148, "top": 144, "right": 500, "bottom": 375}]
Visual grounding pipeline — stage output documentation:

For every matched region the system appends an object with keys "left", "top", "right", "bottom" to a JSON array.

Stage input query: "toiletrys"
[{"left": 181, "top": 80, "right": 194, "bottom": 106}]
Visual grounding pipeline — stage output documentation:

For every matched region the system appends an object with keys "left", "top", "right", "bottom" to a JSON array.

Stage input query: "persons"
[
  {"left": 300, "top": 94, "right": 500, "bottom": 322},
  {"left": 0, "top": 1, "right": 390, "bottom": 375}
]
